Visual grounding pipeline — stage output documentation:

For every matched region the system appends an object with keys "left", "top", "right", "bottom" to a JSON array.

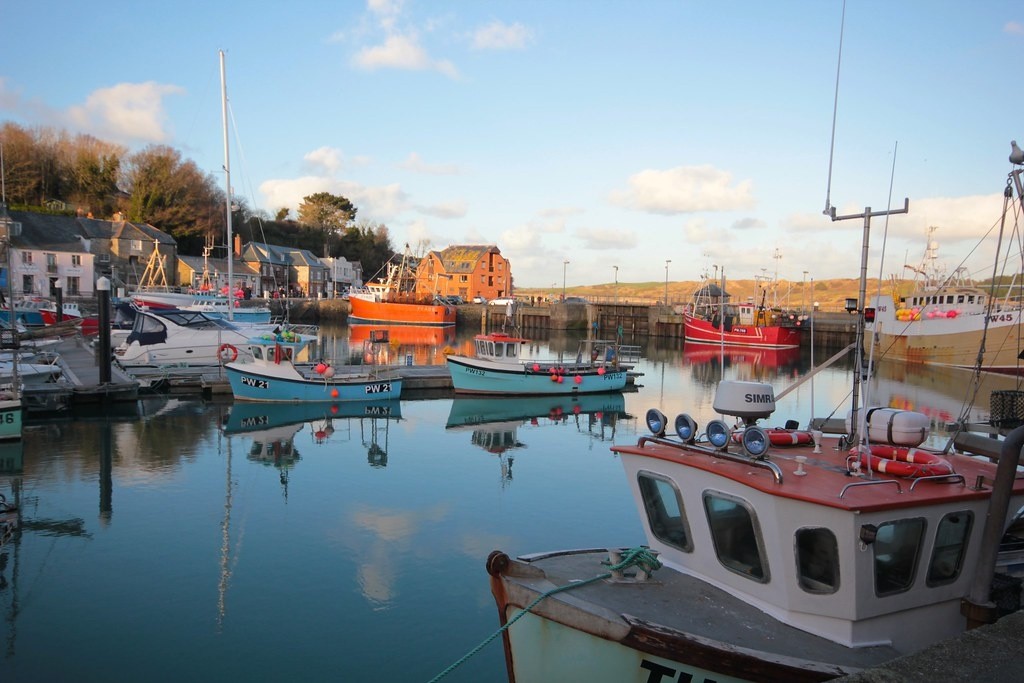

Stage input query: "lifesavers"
[
  {"left": 732, "top": 429, "right": 811, "bottom": 444},
  {"left": 491, "top": 332, "right": 508, "bottom": 338},
  {"left": 848, "top": 443, "right": 955, "bottom": 481},
  {"left": 217, "top": 344, "right": 237, "bottom": 363}
]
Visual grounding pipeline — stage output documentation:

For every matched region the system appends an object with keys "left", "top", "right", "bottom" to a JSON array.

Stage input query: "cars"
[
  {"left": 488, "top": 297, "right": 518, "bottom": 306},
  {"left": 548, "top": 297, "right": 592, "bottom": 307},
  {"left": 473, "top": 296, "right": 485, "bottom": 304},
  {"left": 444, "top": 295, "right": 463, "bottom": 305}
]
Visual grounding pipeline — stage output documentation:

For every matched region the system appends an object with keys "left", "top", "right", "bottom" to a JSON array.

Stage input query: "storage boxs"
[{"left": 845, "top": 406, "right": 931, "bottom": 444}]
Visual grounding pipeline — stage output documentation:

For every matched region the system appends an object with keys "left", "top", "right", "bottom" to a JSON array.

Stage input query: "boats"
[
  {"left": 485, "top": 2, "right": 1023, "bottom": 683},
  {"left": 224, "top": 262, "right": 404, "bottom": 401},
  {"left": 348, "top": 242, "right": 457, "bottom": 323},
  {"left": 0, "top": 295, "right": 98, "bottom": 384},
  {"left": 110, "top": 50, "right": 322, "bottom": 367},
  {"left": 447, "top": 258, "right": 627, "bottom": 395},
  {"left": 684, "top": 255, "right": 805, "bottom": 346},
  {"left": 863, "top": 141, "right": 1024, "bottom": 369}
]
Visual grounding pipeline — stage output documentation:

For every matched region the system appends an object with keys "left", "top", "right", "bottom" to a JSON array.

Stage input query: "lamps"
[{"left": 638, "top": 407, "right": 784, "bottom": 484}]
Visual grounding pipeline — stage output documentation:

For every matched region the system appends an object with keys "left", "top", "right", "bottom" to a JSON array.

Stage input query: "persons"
[
  {"left": 244, "top": 285, "right": 253, "bottom": 301},
  {"left": 606, "top": 346, "right": 616, "bottom": 361}
]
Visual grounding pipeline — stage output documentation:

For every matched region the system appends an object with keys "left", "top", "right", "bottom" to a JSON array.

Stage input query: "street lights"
[
  {"left": 612, "top": 266, "right": 618, "bottom": 304},
  {"left": 563, "top": 259, "right": 570, "bottom": 298},
  {"left": 803, "top": 271, "right": 808, "bottom": 313},
  {"left": 666, "top": 259, "right": 671, "bottom": 305}
]
